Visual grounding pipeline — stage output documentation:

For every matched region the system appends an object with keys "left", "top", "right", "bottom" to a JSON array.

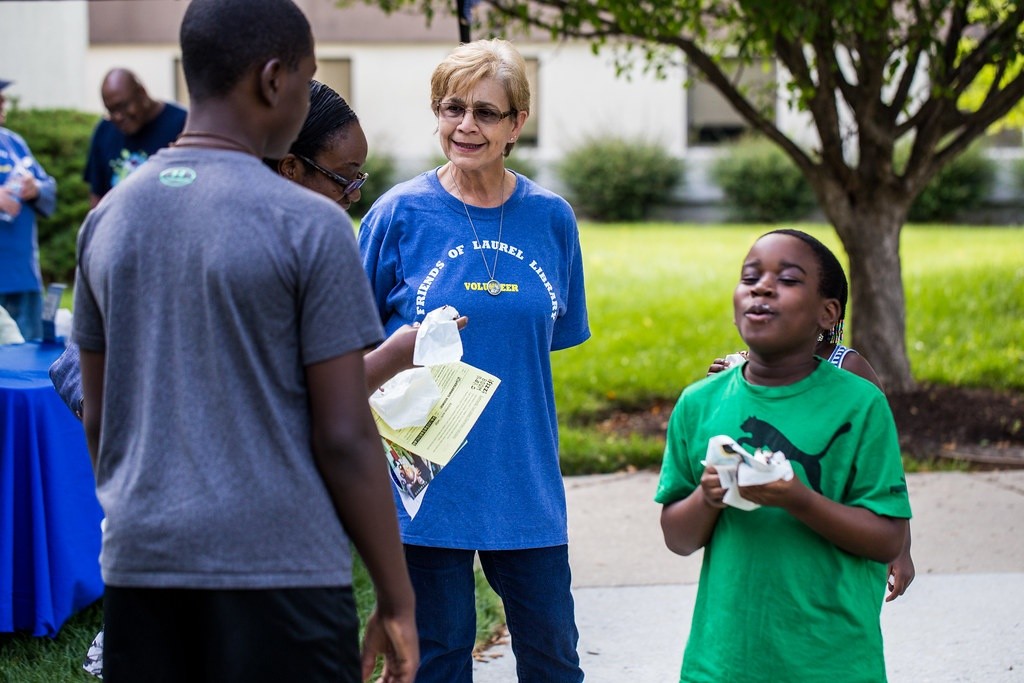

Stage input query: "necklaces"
[
  {"left": 177, "top": 130, "right": 255, "bottom": 152},
  {"left": 450, "top": 165, "right": 509, "bottom": 295}
]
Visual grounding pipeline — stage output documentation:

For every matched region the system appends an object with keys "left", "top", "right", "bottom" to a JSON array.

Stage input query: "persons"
[
  {"left": 354, "top": 38, "right": 593, "bottom": 683},
  {"left": 69, "top": 0, "right": 423, "bottom": 683},
  {"left": 79, "top": 67, "right": 189, "bottom": 211},
  {"left": 652, "top": 228, "right": 912, "bottom": 682},
  {"left": 2, "top": 79, "right": 55, "bottom": 352},
  {"left": 704, "top": 306, "right": 915, "bottom": 604},
  {"left": 260, "top": 81, "right": 374, "bottom": 205}
]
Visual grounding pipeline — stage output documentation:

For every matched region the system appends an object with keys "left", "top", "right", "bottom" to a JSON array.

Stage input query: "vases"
[{"left": 987, "top": 147, "right": 1024, "bottom": 208}]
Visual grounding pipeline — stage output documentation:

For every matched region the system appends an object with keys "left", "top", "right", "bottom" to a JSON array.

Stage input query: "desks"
[{"left": 0, "top": 334, "right": 107, "bottom": 640}]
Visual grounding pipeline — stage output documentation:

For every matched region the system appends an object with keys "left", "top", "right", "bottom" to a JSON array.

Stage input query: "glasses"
[
  {"left": 436, "top": 98, "right": 515, "bottom": 123},
  {"left": 296, "top": 154, "right": 370, "bottom": 193}
]
